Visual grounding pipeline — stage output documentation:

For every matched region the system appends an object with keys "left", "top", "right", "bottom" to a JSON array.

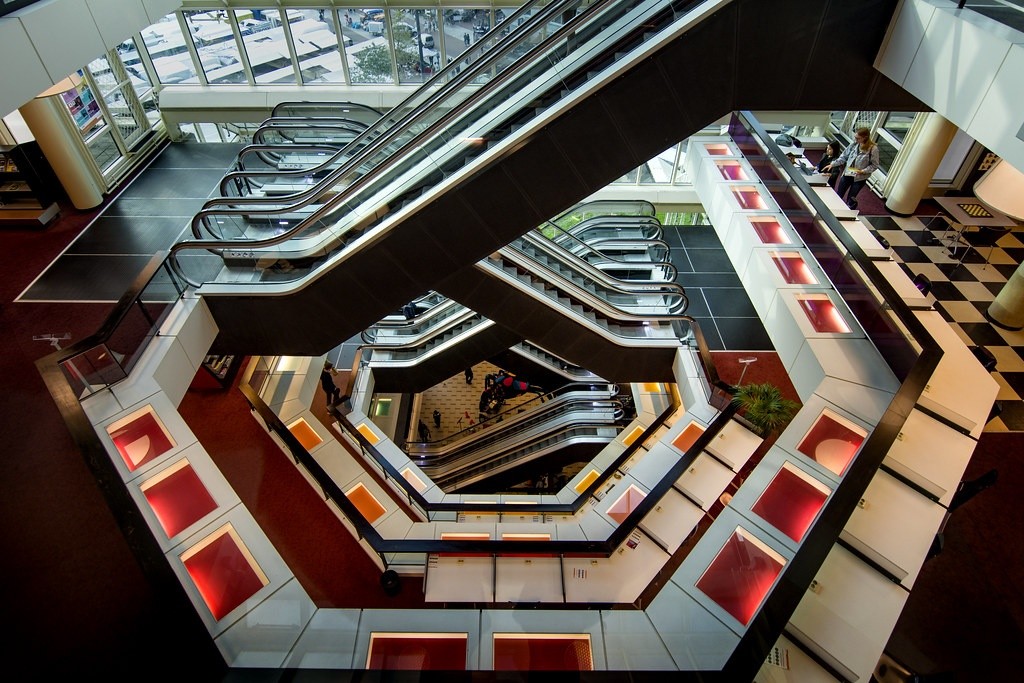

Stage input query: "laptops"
[{"left": 799, "top": 160, "right": 812, "bottom": 176}]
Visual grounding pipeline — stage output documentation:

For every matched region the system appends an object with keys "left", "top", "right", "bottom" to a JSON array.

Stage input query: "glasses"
[{"left": 854, "top": 137, "right": 862, "bottom": 141}]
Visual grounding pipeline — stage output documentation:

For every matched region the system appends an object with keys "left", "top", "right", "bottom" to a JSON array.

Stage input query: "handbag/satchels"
[{"left": 429, "top": 433, "right": 431, "bottom": 438}]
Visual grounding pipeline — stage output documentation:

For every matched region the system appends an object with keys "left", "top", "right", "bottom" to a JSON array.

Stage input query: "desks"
[
  {"left": 933, "top": 197, "right": 1019, "bottom": 258},
  {"left": 424, "top": 133, "right": 1000, "bottom": 683}
]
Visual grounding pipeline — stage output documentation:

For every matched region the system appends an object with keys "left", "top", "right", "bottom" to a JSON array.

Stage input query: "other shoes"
[
  {"left": 467, "top": 382, "right": 472, "bottom": 385},
  {"left": 327, "top": 412, "right": 332, "bottom": 414}
]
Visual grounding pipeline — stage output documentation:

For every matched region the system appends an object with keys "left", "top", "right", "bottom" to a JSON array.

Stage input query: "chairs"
[
  {"left": 923, "top": 190, "right": 970, "bottom": 245},
  {"left": 942, "top": 227, "right": 1011, "bottom": 270}
]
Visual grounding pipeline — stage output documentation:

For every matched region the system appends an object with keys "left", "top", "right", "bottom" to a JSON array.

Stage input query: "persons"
[
  {"left": 320, "top": 360, "right": 341, "bottom": 415},
  {"left": 815, "top": 127, "right": 880, "bottom": 206},
  {"left": 418, "top": 419, "right": 431, "bottom": 442},
  {"left": 403, "top": 301, "right": 420, "bottom": 334},
  {"left": 318, "top": 7, "right": 486, "bottom": 78},
  {"left": 433, "top": 410, "right": 441, "bottom": 428},
  {"left": 464, "top": 367, "right": 473, "bottom": 385}
]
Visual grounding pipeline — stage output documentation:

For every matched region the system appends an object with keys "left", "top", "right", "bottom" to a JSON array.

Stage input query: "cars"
[
  {"left": 413, "top": 33, "right": 435, "bottom": 50},
  {"left": 383, "top": 22, "right": 418, "bottom": 38}
]
